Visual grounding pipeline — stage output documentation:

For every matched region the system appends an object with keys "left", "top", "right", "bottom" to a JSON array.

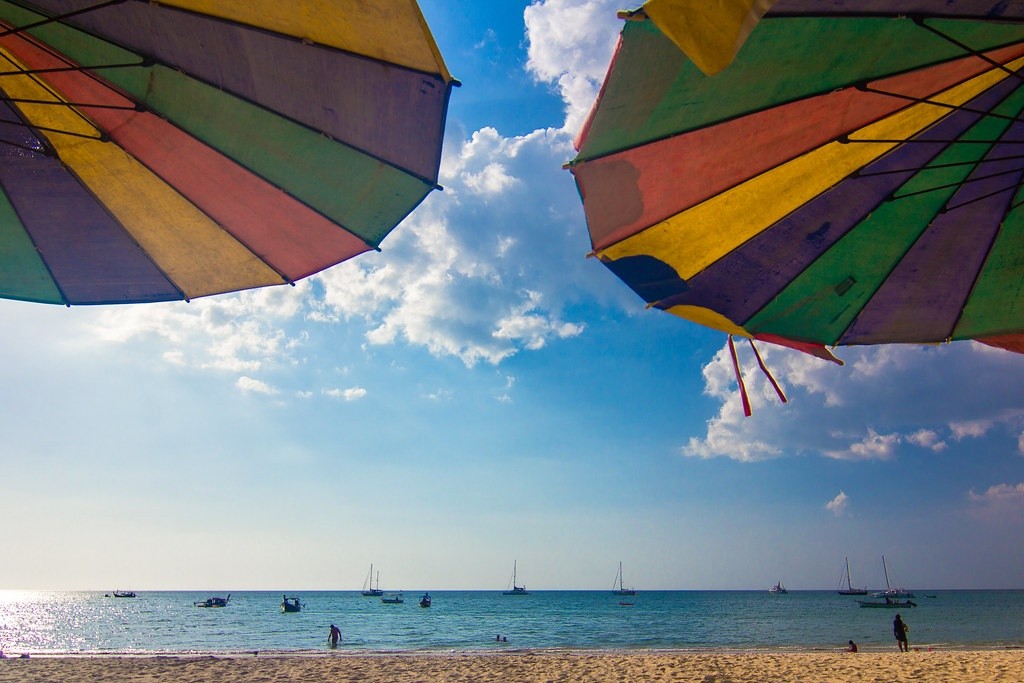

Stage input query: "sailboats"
[
  {"left": 610, "top": 561, "right": 634, "bottom": 595},
  {"left": 360, "top": 563, "right": 383, "bottom": 597},
  {"left": 837, "top": 557, "right": 867, "bottom": 595},
  {"left": 871, "top": 555, "right": 915, "bottom": 598},
  {"left": 502, "top": 560, "right": 529, "bottom": 595}
]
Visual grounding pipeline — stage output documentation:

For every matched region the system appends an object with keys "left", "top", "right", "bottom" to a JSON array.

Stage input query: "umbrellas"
[
  {"left": 561, "top": 0, "right": 1023, "bottom": 367},
  {"left": 0, "top": 0, "right": 462, "bottom": 307}
]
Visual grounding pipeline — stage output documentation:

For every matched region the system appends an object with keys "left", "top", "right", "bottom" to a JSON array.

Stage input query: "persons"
[
  {"left": 497, "top": 634, "right": 500, "bottom": 640},
  {"left": 327, "top": 624, "right": 342, "bottom": 645},
  {"left": 502, "top": 637, "right": 506, "bottom": 642},
  {"left": 844, "top": 640, "right": 858, "bottom": 653},
  {"left": 893, "top": 613, "right": 910, "bottom": 652}
]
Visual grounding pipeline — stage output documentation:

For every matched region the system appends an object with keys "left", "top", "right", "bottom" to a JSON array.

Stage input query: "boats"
[
  {"left": 113, "top": 590, "right": 136, "bottom": 598},
  {"left": 389, "top": 593, "right": 403, "bottom": 597},
  {"left": 418, "top": 592, "right": 431, "bottom": 607},
  {"left": 194, "top": 594, "right": 231, "bottom": 607},
  {"left": 415, "top": 595, "right": 431, "bottom": 599},
  {"left": 381, "top": 597, "right": 404, "bottom": 603},
  {"left": 619, "top": 602, "right": 633, "bottom": 605},
  {"left": 855, "top": 600, "right": 917, "bottom": 608},
  {"left": 279, "top": 595, "right": 305, "bottom": 612},
  {"left": 924, "top": 594, "right": 936, "bottom": 598},
  {"left": 769, "top": 581, "right": 789, "bottom": 594}
]
